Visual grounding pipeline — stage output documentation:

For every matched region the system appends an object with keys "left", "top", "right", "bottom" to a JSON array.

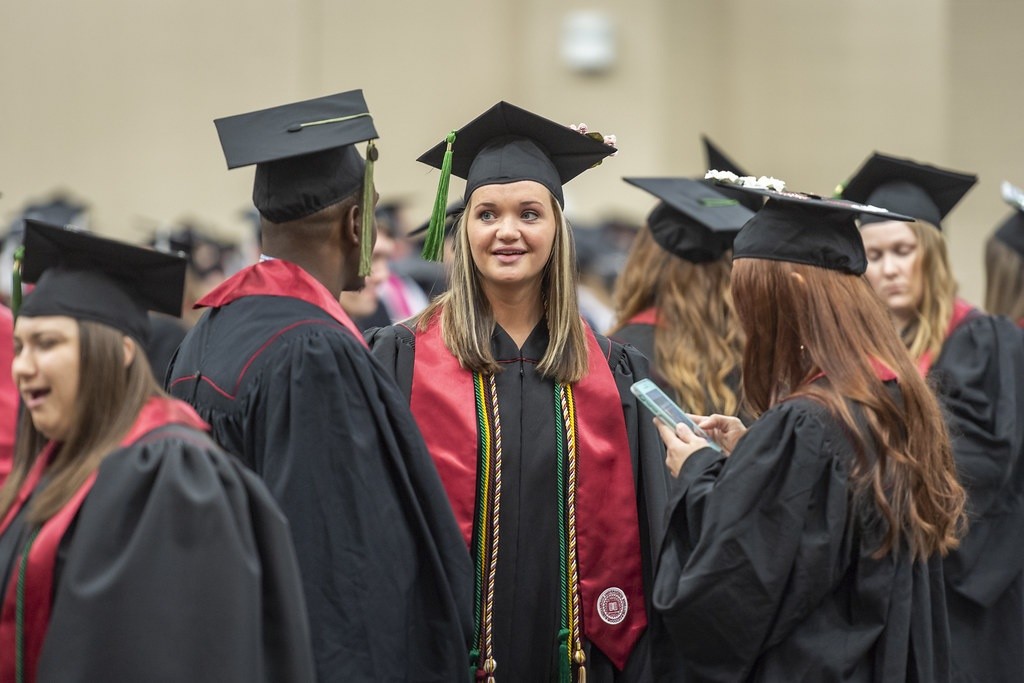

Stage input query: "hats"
[
  {"left": 991, "top": 183, "right": 1024, "bottom": 254},
  {"left": 11, "top": 218, "right": 189, "bottom": 354},
  {"left": 415, "top": 98, "right": 618, "bottom": 264},
  {"left": 702, "top": 135, "right": 750, "bottom": 178},
  {"left": 706, "top": 179, "right": 918, "bottom": 276},
  {"left": 622, "top": 176, "right": 765, "bottom": 263},
  {"left": 830, "top": 153, "right": 979, "bottom": 232},
  {"left": 212, "top": 88, "right": 379, "bottom": 279}
]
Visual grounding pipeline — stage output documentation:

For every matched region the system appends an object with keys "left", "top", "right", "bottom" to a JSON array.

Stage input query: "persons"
[
  {"left": 356, "top": 98, "right": 673, "bottom": 682},
  {"left": 826, "top": 147, "right": 1024, "bottom": 681},
  {"left": 157, "top": 90, "right": 484, "bottom": 683},
  {"left": 0, "top": 147, "right": 654, "bottom": 368},
  {"left": 652, "top": 180, "right": 968, "bottom": 683},
  {"left": 974, "top": 182, "right": 1024, "bottom": 329},
  {"left": 0, "top": 216, "right": 315, "bottom": 683}
]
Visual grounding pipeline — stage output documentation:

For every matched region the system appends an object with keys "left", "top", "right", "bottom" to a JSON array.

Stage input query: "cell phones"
[{"left": 629, "top": 377, "right": 723, "bottom": 454}]
